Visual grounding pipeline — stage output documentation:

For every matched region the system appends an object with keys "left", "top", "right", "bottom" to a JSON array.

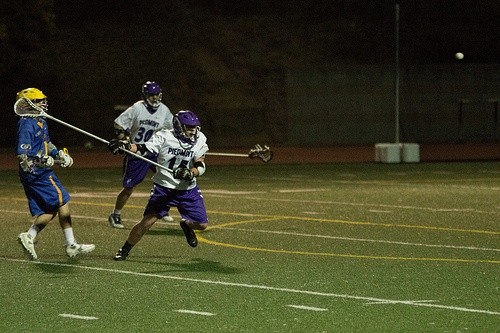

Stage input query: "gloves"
[
  {"left": 56, "top": 147, "right": 74, "bottom": 168},
  {"left": 108, "top": 139, "right": 131, "bottom": 156},
  {"left": 36, "top": 150, "right": 54, "bottom": 168},
  {"left": 178, "top": 168, "right": 195, "bottom": 183}
]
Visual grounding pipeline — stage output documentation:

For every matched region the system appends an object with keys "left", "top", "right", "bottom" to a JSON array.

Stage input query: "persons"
[
  {"left": 108, "top": 80, "right": 174, "bottom": 228},
  {"left": 15, "top": 87, "right": 96, "bottom": 262},
  {"left": 110, "top": 110, "right": 209, "bottom": 262}
]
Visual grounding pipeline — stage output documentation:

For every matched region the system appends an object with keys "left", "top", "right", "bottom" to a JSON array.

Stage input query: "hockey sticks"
[
  {"left": 203, "top": 144, "right": 273, "bottom": 164},
  {"left": 13, "top": 97, "right": 174, "bottom": 174}
]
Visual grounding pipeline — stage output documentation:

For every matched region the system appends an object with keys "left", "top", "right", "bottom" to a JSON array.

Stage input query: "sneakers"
[
  {"left": 162, "top": 215, "right": 174, "bottom": 222},
  {"left": 108, "top": 213, "right": 124, "bottom": 229},
  {"left": 64, "top": 243, "right": 96, "bottom": 257},
  {"left": 17, "top": 232, "right": 37, "bottom": 259},
  {"left": 113, "top": 247, "right": 129, "bottom": 261},
  {"left": 180, "top": 219, "right": 198, "bottom": 247}
]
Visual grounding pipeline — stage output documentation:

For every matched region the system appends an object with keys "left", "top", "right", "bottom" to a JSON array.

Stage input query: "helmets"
[
  {"left": 141, "top": 81, "right": 162, "bottom": 113},
  {"left": 172, "top": 110, "right": 201, "bottom": 145},
  {"left": 16, "top": 87, "right": 48, "bottom": 119}
]
